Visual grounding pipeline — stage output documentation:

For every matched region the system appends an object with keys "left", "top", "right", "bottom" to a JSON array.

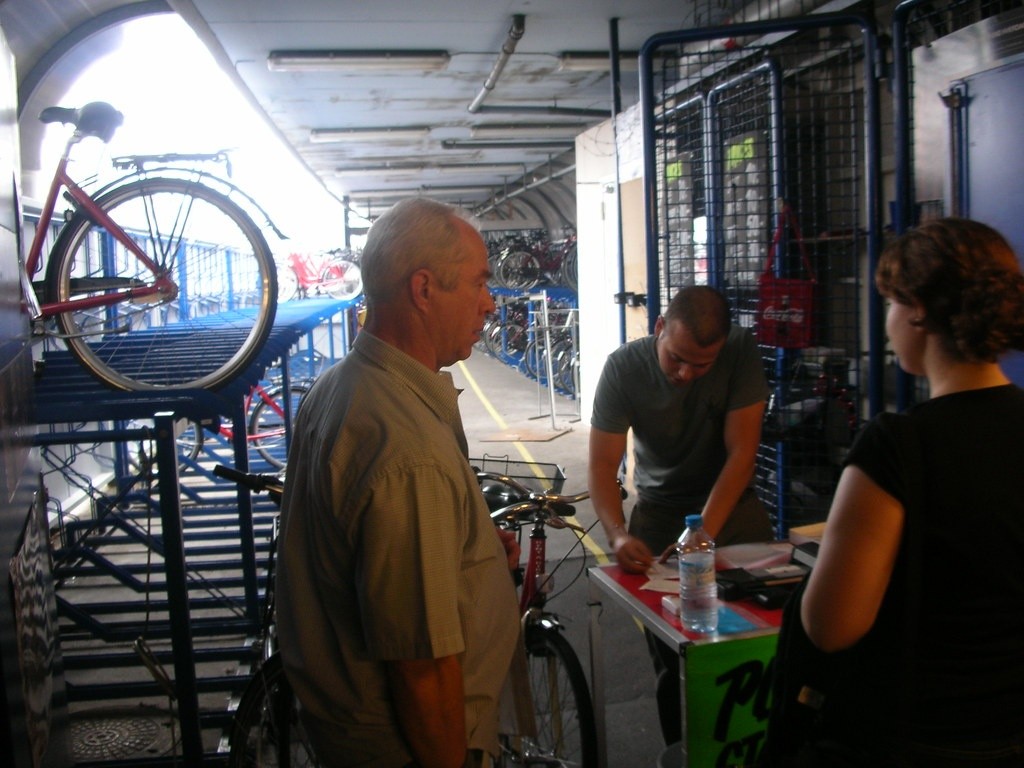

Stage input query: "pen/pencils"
[{"left": 747, "top": 577, "right": 804, "bottom": 588}]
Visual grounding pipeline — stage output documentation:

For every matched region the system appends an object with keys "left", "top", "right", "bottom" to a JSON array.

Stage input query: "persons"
[
  {"left": 799, "top": 217, "right": 1024, "bottom": 768},
  {"left": 277, "top": 200, "right": 501, "bottom": 768},
  {"left": 588, "top": 285, "right": 774, "bottom": 747}
]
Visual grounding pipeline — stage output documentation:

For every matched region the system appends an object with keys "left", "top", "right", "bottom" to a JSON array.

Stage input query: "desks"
[{"left": 586, "top": 548, "right": 785, "bottom": 768}]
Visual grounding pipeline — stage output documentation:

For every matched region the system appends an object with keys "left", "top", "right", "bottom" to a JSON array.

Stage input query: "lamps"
[
  {"left": 558, "top": 50, "right": 678, "bottom": 73},
  {"left": 469, "top": 124, "right": 586, "bottom": 140},
  {"left": 335, "top": 166, "right": 423, "bottom": 175},
  {"left": 438, "top": 163, "right": 525, "bottom": 173},
  {"left": 311, "top": 126, "right": 431, "bottom": 144},
  {"left": 266, "top": 50, "right": 450, "bottom": 71}
]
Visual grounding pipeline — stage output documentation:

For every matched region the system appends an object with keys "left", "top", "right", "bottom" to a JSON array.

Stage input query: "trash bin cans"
[{"left": 657, "top": 741, "right": 683, "bottom": 768}]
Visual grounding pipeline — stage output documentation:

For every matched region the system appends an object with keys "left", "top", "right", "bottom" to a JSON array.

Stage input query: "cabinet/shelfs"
[
  {"left": 477, "top": 285, "right": 579, "bottom": 399},
  {"left": 0, "top": 194, "right": 369, "bottom": 768}
]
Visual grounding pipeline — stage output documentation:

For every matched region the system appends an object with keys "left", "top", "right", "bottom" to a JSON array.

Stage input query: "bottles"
[{"left": 677, "top": 513, "right": 718, "bottom": 633}]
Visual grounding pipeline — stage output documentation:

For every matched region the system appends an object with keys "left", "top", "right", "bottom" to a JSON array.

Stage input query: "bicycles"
[
  {"left": 479, "top": 224, "right": 581, "bottom": 397},
  {"left": 192, "top": 463, "right": 314, "bottom": 768},
  {"left": 467, "top": 453, "right": 626, "bottom": 768},
  {"left": 15, "top": 101, "right": 289, "bottom": 392},
  {"left": 276, "top": 241, "right": 365, "bottom": 304},
  {"left": 124, "top": 356, "right": 312, "bottom": 475}
]
